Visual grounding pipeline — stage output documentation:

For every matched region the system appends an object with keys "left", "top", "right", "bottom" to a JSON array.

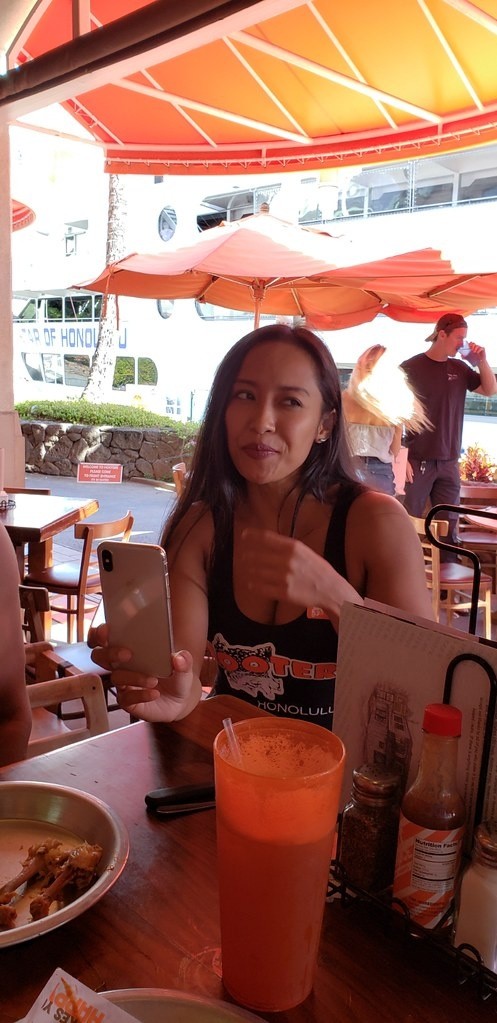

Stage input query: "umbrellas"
[{"left": 68, "top": 200, "right": 497, "bottom": 332}]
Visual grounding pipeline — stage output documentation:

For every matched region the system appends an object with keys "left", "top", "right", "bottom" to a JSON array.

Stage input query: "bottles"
[
  {"left": 334, "top": 764, "right": 406, "bottom": 895},
  {"left": 451, "top": 822, "right": 497, "bottom": 975},
  {"left": 392, "top": 703, "right": 467, "bottom": 937}
]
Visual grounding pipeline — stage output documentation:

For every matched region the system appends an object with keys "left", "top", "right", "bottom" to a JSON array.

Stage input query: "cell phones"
[{"left": 97, "top": 541, "right": 175, "bottom": 680}]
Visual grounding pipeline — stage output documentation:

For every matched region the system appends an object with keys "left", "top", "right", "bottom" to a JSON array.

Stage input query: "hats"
[{"left": 425, "top": 314, "right": 468, "bottom": 341}]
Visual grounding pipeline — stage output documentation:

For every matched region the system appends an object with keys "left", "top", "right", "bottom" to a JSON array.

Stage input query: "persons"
[
  {"left": 340, "top": 343, "right": 432, "bottom": 501},
  {"left": 398, "top": 313, "right": 497, "bottom": 614},
  {"left": 0, "top": 519, "right": 32, "bottom": 768},
  {"left": 87, "top": 323, "right": 440, "bottom": 733}
]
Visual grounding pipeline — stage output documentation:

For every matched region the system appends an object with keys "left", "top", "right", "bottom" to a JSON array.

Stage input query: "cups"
[
  {"left": 457, "top": 341, "right": 482, "bottom": 367},
  {"left": 214, "top": 716, "right": 347, "bottom": 1012}
]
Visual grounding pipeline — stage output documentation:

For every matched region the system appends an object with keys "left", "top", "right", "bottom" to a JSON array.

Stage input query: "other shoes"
[{"left": 440, "top": 596, "right": 471, "bottom": 616}]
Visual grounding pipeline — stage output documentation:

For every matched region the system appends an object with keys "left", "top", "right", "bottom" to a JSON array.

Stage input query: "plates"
[{"left": 13, "top": 988, "right": 270, "bottom": 1023}]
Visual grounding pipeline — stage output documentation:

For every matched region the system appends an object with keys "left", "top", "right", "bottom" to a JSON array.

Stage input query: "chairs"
[
  {"left": 0, "top": 487, "right": 135, "bottom": 644},
  {"left": 409, "top": 484, "right": 497, "bottom": 642},
  {"left": 19, "top": 585, "right": 139, "bottom": 732}
]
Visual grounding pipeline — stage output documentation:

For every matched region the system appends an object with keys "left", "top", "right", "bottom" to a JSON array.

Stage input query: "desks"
[
  {"left": 0, "top": 493, "right": 99, "bottom": 642},
  {"left": 463, "top": 511, "right": 497, "bottom": 533},
  {"left": 0, "top": 693, "right": 497, "bottom": 1023}
]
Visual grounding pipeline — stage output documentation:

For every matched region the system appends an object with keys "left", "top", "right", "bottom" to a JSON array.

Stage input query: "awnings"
[{"left": 10, "top": 1, "right": 497, "bottom": 172}]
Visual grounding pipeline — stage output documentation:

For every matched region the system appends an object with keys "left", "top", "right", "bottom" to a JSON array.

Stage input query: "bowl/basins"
[{"left": 0, "top": 781, "right": 130, "bottom": 949}]
